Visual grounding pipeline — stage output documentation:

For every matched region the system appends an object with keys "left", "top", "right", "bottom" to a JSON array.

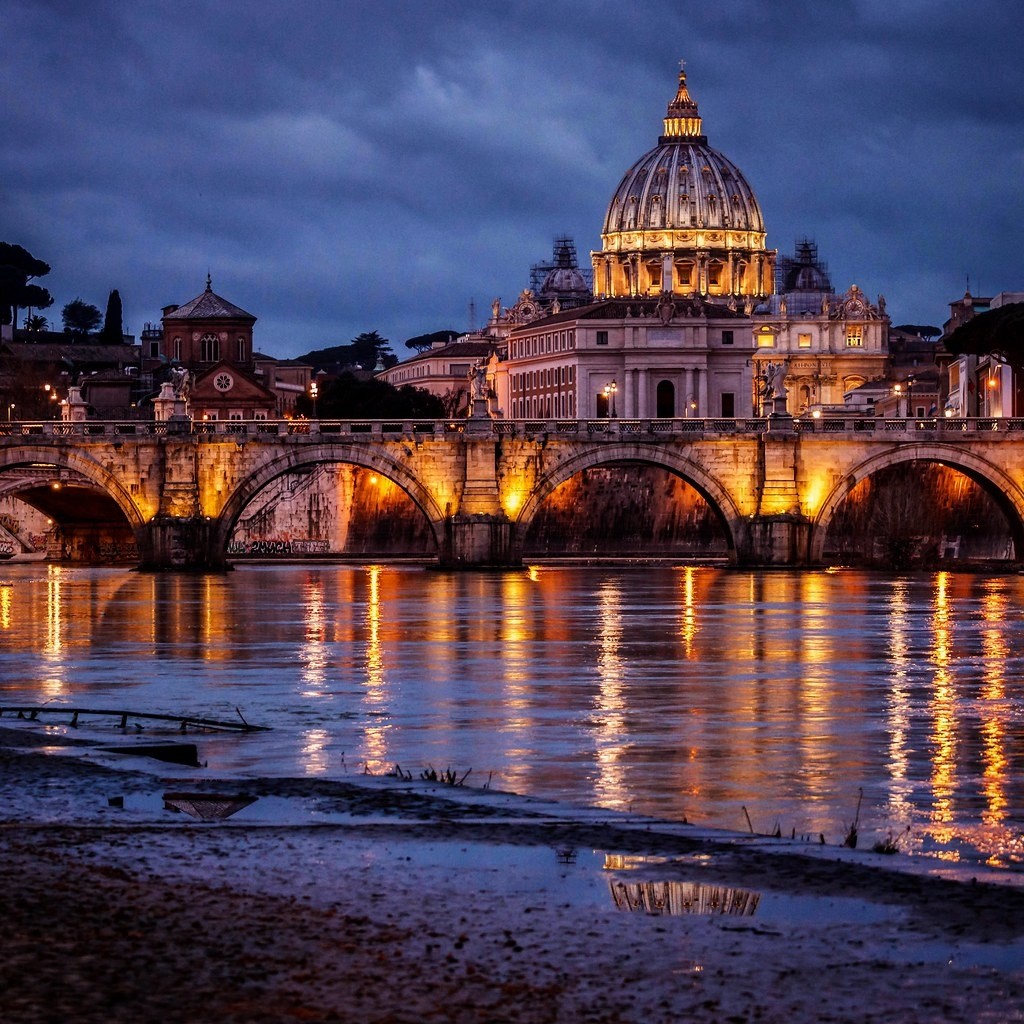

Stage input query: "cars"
[{"left": 412, "top": 422, "right": 456, "bottom": 433}]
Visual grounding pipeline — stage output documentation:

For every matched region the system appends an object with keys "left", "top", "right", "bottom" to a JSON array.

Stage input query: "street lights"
[
  {"left": 309, "top": 381, "right": 319, "bottom": 419},
  {"left": 746, "top": 359, "right": 771, "bottom": 418},
  {"left": 611, "top": 379, "right": 617, "bottom": 418},
  {"left": 907, "top": 378, "right": 913, "bottom": 417},
  {"left": 604, "top": 383, "right": 611, "bottom": 418},
  {"left": 895, "top": 384, "right": 901, "bottom": 417},
  {"left": 685, "top": 393, "right": 696, "bottom": 418},
  {"left": 45, "top": 380, "right": 51, "bottom": 420},
  {"left": 7, "top": 400, "right": 16, "bottom": 421}
]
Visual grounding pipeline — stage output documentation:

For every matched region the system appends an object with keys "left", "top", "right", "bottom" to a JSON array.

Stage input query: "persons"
[
  {"left": 172, "top": 367, "right": 190, "bottom": 399},
  {"left": 878, "top": 294, "right": 886, "bottom": 309},
  {"left": 754, "top": 360, "right": 788, "bottom": 398},
  {"left": 835, "top": 301, "right": 845, "bottom": 320},
  {"left": 470, "top": 360, "right": 488, "bottom": 404},
  {"left": 727, "top": 294, "right": 737, "bottom": 311},
  {"left": 550, "top": 297, "right": 561, "bottom": 314},
  {"left": 821, "top": 293, "right": 831, "bottom": 314},
  {"left": 742, "top": 294, "right": 754, "bottom": 315}
]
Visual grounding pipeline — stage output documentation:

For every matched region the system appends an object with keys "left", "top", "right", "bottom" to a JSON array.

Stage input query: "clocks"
[{"left": 847, "top": 300, "right": 864, "bottom": 315}]
